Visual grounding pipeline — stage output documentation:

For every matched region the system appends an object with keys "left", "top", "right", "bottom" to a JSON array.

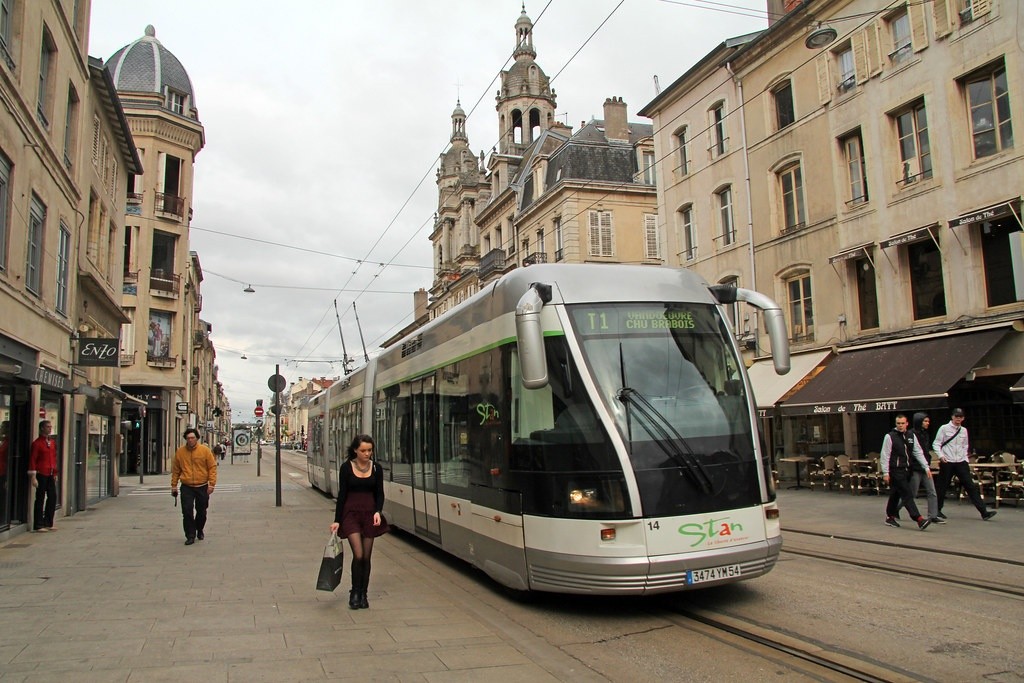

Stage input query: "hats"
[{"left": 952, "top": 408, "right": 965, "bottom": 416}]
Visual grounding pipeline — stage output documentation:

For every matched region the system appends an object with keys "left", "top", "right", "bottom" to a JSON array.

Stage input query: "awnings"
[{"left": 732, "top": 319, "right": 1024, "bottom": 419}]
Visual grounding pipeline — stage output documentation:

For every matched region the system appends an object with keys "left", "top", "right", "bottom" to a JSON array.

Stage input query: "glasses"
[{"left": 955, "top": 415, "right": 964, "bottom": 418}]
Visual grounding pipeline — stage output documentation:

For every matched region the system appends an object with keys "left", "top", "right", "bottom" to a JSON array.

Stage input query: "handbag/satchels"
[{"left": 315, "top": 530, "right": 344, "bottom": 592}]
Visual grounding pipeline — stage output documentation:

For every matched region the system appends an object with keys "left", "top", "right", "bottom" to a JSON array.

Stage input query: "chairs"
[{"left": 810, "top": 450, "right": 1024, "bottom": 507}]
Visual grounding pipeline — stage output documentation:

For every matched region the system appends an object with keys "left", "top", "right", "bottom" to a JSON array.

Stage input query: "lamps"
[
  {"left": 241, "top": 354, "right": 247, "bottom": 359},
  {"left": 243, "top": 285, "right": 255, "bottom": 292},
  {"left": 805, "top": 22, "right": 837, "bottom": 50}
]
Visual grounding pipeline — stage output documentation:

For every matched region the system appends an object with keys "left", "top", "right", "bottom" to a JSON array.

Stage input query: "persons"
[
  {"left": 935, "top": 408, "right": 997, "bottom": 521},
  {"left": 879, "top": 413, "right": 933, "bottom": 531},
  {"left": 151, "top": 322, "right": 164, "bottom": 358},
  {"left": 27, "top": 420, "right": 58, "bottom": 532},
  {"left": 893, "top": 413, "right": 946, "bottom": 524},
  {"left": 292, "top": 439, "right": 307, "bottom": 451},
  {"left": 171, "top": 429, "right": 249, "bottom": 545},
  {"left": 329, "top": 435, "right": 390, "bottom": 609},
  {"left": 0, "top": 420, "right": 10, "bottom": 526}
]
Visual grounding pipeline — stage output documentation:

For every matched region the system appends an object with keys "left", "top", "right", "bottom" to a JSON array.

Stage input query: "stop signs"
[{"left": 254, "top": 407, "right": 264, "bottom": 416}]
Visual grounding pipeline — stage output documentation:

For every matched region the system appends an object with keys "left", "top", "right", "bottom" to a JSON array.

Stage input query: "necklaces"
[{"left": 354, "top": 459, "right": 370, "bottom": 473}]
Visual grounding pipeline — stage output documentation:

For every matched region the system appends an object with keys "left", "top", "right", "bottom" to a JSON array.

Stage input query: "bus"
[
  {"left": 255, "top": 436, "right": 262, "bottom": 443},
  {"left": 306, "top": 263, "right": 791, "bottom": 594}
]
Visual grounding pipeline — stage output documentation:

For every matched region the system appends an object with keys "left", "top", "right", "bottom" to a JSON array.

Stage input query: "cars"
[
  {"left": 259, "top": 440, "right": 273, "bottom": 445},
  {"left": 280, "top": 441, "right": 302, "bottom": 450}
]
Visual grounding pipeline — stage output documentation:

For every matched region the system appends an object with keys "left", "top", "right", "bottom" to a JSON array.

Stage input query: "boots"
[{"left": 349, "top": 570, "right": 369, "bottom": 610}]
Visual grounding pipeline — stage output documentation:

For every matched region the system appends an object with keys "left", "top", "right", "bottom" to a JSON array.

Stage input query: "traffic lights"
[{"left": 133, "top": 421, "right": 139, "bottom": 430}]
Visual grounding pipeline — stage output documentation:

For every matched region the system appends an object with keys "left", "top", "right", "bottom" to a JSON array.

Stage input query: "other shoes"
[
  {"left": 197, "top": 529, "right": 205, "bottom": 540},
  {"left": 918, "top": 519, "right": 931, "bottom": 530},
  {"left": 981, "top": 511, "right": 997, "bottom": 521},
  {"left": 185, "top": 538, "right": 195, "bottom": 545},
  {"left": 885, "top": 517, "right": 900, "bottom": 528},
  {"left": 937, "top": 512, "right": 948, "bottom": 520},
  {"left": 930, "top": 518, "right": 946, "bottom": 524}
]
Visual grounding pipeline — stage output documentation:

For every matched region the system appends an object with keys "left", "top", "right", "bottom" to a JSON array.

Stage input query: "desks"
[
  {"left": 850, "top": 460, "right": 876, "bottom": 492},
  {"left": 971, "top": 463, "right": 1021, "bottom": 507},
  {"left": 780, "top": 455, "right": 814, "bottom": 490}
]
